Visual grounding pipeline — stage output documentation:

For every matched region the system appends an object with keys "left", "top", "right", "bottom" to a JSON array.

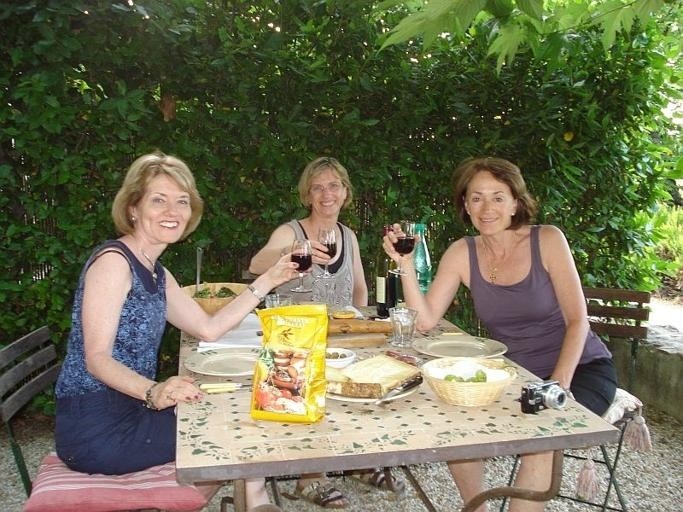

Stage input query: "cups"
[
  {"left": 389, "top": 299, "right": 416, "bottom": 349},
  {"left": 265, "top": 294, "right": 291, "bottom": 309}
]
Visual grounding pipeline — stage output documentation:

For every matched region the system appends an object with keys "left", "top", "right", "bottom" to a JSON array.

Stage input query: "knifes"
[{"left": 374, "top": 376, "right": 421, "bottom": 406}]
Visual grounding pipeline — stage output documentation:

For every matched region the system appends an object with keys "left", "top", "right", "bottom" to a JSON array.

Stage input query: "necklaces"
[
  {"left": 137, "top": 243, "right": 159, "bottom": 279},
  {"left": 483, "top": 242, "right": 510, "bottom": 284}
]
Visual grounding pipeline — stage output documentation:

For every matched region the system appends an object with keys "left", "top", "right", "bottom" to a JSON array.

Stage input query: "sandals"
[
  {"left": 343, "top": 468, "right": 396, "bottom": 490},
  {"left": 294, "top": 477, "right": 349, "bottom": 508}
]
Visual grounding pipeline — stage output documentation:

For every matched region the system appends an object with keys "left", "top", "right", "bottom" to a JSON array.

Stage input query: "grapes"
[
  {"left": 445, "top": 371, "right": 488, "bottom": 383},
  {"left": 325, "top": 353, "right": 345, "bottom": 359}
]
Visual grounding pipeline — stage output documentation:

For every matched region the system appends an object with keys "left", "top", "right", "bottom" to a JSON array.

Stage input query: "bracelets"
[
  {"left": 145, "top": 382, "right": 161, "bottom": 411},
  {"left": 280, "top": 246, "right": 286, "bottom": 258},
  {"left": 248, "top": 285, "right": 265, "bottom": 302}
]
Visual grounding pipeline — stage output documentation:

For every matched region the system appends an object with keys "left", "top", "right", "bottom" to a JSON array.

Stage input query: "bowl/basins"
[{"left": 327, "top": 348, "right": 357, "bottom": 369}]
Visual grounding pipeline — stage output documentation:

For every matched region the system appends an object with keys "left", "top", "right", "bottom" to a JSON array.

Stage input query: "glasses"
[{"left": 308, "top": 182, "right": 343, "bottom": 191}]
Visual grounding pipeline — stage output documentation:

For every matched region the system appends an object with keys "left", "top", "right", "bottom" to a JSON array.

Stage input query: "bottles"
[{"left": 375, "top": 223, "right": 432, "bottom": 317}]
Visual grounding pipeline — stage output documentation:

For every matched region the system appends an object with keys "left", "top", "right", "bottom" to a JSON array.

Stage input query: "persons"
[
  {"left": 248, "top": 157, "right": 406, "bottom": 508},
  {"left": 381, "top": 158, "right": 619, "bottom": 512},
  {"left": 56, "top": 152, "right": 310, "bottom": 511}
]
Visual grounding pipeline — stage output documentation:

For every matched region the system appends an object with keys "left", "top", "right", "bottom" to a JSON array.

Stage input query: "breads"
[
  {"left": 323, "top": 363, "right": 348, "bottom": 393},
  {"left": 344, "top": 354, "right": 422, "bottom": 399},
  {"left": 327, "top": 318, "right": 394, "bottom": 333},
  {"left": 333, "top": 306, "right": 356, "bottom": 318}
]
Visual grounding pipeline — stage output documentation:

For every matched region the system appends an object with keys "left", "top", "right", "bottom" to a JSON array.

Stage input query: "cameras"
[{"left": 521, "top": 380, "right": 568, "bottom": 414}]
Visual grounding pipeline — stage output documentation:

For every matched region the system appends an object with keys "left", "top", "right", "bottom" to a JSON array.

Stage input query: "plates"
[
  {"left": 184, "top": 347, "right": 257, "bottom": 377},
  {"left": 413, "top": 335, "right": 508, "bottom": 360},
  {"left": 324, "top": 385, "right": 423, "bottom": 405}
]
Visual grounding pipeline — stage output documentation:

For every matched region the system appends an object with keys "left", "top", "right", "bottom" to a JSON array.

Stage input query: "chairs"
[
  {"left": 0, "top": 326, "right": 208, "bottom": 512},
  {"left": 501, "top": 288, "right": 653, "bottom": 512}
]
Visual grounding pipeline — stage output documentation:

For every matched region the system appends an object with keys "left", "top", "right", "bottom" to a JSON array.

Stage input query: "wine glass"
[
  {"left": 317, "top": 227, "right": 337, "bottom": 279},
  {"left": 290, "top": 240, "right": 311, "bottom": 293}
]
